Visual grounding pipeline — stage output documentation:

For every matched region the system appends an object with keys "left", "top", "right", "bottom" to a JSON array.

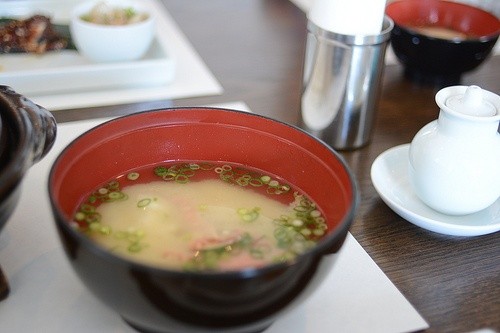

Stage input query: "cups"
[{"left": 299, "top": 15, "right": 394, "bottom": 152}]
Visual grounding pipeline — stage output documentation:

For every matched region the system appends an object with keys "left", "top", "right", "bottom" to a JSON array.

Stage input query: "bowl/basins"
[
  {"left": 70, "top": 0, "right": 160, "bottom": 63},
  {"left": 386, "top": 0, "right": 500, "bottom": 90},
  {"left": 46, "top": 107, "right": 361, "bottom": 333}
]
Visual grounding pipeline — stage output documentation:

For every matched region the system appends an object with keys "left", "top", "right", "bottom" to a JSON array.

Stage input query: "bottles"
[{"left": 412, "top": 85, "right": 500, "bottom": 215}]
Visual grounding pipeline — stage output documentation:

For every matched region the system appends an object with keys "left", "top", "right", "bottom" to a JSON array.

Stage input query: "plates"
[
  {"left": 0, "top": 1, "right": 224, "bottom": 109},
  {"left": 1, "top": 102, "right": 428, "bottom": 332},
  {"left": 370, "top": 145, "right": 499, "bottom": 238}
]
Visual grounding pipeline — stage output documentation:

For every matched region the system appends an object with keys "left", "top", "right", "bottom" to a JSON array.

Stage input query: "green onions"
[{"left": 73, "top": 161, "right": 328, "bottom": 270}]
